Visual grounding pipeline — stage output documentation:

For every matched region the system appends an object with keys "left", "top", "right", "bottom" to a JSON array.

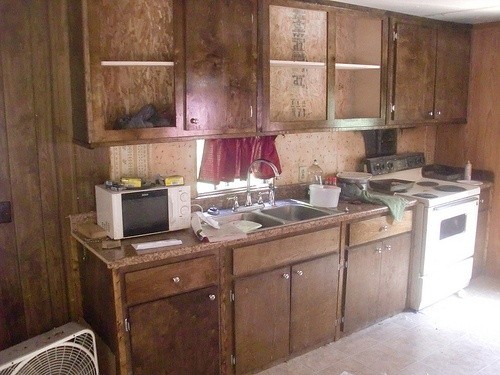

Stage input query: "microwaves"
[{"left": 95, "top": 184, "right": 191, "bottom": 240}]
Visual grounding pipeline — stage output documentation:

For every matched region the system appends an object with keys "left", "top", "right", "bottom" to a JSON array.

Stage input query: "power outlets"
[{"left": 299, "top": 166, "right": 307, "bottom": 182}]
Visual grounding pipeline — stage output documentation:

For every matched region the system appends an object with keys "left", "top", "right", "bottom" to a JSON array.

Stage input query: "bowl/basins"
[{"left": 309, "top": 184, "right": 341, "bottom": 207}]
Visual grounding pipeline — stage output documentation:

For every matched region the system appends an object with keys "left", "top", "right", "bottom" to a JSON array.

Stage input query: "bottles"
[
  {"left": 325, "top": 176, "right": 337, "bottom": 186},
  {"left": 306, "top": 160, "right": 323, "bottom": 200},
  {"left": 464, "top": 161, "right": 472, "bottom": 180}
]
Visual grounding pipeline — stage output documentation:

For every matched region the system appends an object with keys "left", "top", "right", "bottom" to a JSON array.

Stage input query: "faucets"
[{"left": 247, "top": 159, "right": 279, "bottom": 208}]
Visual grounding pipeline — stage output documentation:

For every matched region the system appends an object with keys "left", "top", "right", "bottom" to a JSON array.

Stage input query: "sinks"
[
  {"left": 213, "top": 210, "right": 283, "bottom": 236},
  {"left": 255, "top": 203, "right": 329, "bottom": 221}
]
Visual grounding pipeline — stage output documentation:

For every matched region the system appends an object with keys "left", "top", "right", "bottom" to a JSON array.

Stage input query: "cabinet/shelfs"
[
  {"left": 222, "top": 222, "right": 344, "bottom": 375},
  {"left": 261, "top": 0, "right": 387, "bottom": 136},
  {"left": 339, "top": 206, "right": 416, "bottom": 341},
  {"left": 68, "top": 0, "right": 262, "bottom": 149},
  {"left": 472, "top": 186, "right": 493, "bottom": 278},
  {"left": 77, "top": 241, "right": 230, "bottom": 375},
  {"left": 387, "top": 11, "right": 473, "bottom": 128}
]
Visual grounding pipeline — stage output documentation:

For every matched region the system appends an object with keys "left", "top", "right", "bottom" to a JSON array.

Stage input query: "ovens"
[{"left": 406, "top": 195, "right": 480, "bottom": 311}]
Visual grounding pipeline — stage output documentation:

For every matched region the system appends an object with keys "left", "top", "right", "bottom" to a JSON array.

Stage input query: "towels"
[{"left": 359, "top": 190, "right": 407, "bottom": 223}]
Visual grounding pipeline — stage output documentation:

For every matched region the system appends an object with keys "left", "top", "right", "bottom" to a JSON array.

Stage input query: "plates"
[
  {"left": 336, "top": 171, "right": 372, "bottom": 184},
  {"left": 231, "top": 220, "right": 263, "bottom": 232},
  {"left": 456, "top": 180, "right": 484, "bottom": 185}
]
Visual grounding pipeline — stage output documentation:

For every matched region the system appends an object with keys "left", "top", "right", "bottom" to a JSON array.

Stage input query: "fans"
[{"left": 0, "top": 320, "right": 99, "bottom": 375}]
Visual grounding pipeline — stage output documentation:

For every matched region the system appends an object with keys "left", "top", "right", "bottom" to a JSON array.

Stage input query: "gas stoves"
[{"left": 363, "top": 152, "right": 481, "bottom": 208}]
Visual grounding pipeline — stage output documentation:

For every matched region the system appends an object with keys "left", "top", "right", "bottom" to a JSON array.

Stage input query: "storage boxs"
[
  {"left": 121, "top": 178, "right": 142, "bottom": 188},
  {"left": 165, "top": 176, "right": 184, "bottom": 186}
]
